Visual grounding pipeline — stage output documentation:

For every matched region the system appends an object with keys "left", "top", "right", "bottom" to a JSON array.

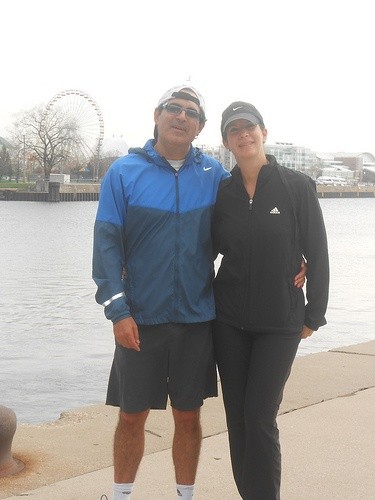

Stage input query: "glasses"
[{"left": 159, "top": 104, "right": 202, "bottom": 121}]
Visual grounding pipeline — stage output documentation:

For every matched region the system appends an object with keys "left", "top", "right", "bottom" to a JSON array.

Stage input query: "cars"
[{"left": 313, "top": 176, "right": 348, "bottom": 186}]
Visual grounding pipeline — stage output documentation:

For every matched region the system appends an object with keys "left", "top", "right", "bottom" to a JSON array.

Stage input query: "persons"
[
  {"left": 121, "top": 100, "right": 332, "bottom": 500},
  {"left": 88, "top": 81, "right": 310, "bottom": 499}
]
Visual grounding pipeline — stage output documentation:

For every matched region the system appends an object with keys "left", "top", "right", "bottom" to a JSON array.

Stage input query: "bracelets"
[{"left": 101, "top": 285, "right": 126, "bottom": 309}]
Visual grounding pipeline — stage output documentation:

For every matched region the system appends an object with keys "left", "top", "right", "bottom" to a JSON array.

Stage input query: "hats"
[
  {"left": 155, "top": 85, "right": 206, "bottom": 118},
  {"left": 220, "top": 100, "right": 264, "bottom": 137}
]
